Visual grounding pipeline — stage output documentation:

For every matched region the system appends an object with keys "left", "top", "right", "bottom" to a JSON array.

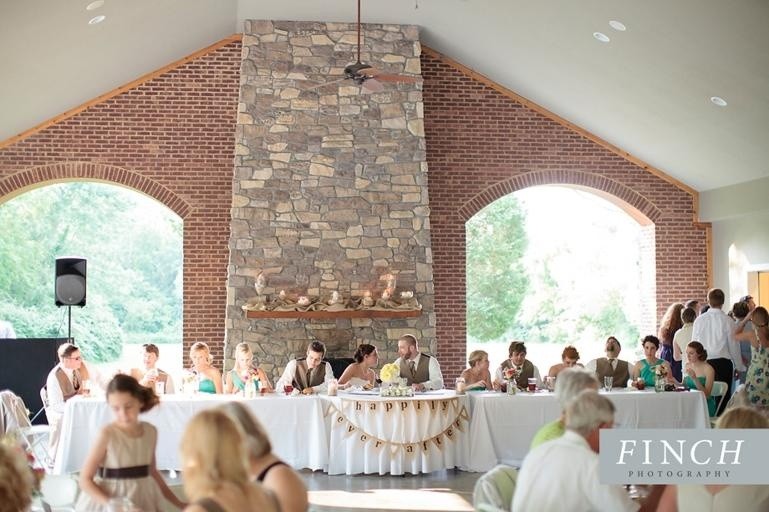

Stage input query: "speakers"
[{"left": 55, "top": 258, "right": 87, "bottom": 306}]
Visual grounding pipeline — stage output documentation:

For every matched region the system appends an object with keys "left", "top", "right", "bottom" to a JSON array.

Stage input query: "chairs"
[
  {"left": 0, "top": 388, "right": 55, "bottom": 472},
  {"left": 705, "top": 381, "right": 728, "bottom": 417}
]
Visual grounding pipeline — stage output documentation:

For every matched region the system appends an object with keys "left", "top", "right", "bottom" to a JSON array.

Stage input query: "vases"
[
  {"left": 244, "top": 382, "right": 256, "bottom": 400},
  {"left": 655, "top": 377, "right": 666, "bottom": 392},
  {"left": 507, "top": 381, "right": 517, "bottom": 394}
]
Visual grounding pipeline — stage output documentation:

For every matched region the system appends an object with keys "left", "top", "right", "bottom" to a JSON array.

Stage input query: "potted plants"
[{"left": 48, "top": 389, "right": 713, "bottom": 485}]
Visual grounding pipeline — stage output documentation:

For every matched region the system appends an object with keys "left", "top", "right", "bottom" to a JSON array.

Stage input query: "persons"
[
  {"left": 495, "top": 340, "right": 543, "bottom": 392},
  {"left": 527, "top": 367, "right": 601, "bottom": 451},
  {"left": 335, "top": 343, "right": 380, "bottom": 392},
  {"left": 591, "top": 335, "right": 636, "bottom": 389},
  {"left": 1, "top": 334, "right": 337, "bottom": 512},
  {"left": 634, "top": 287, "right": 769, "bottom": 511},
  {"left": 389, "top": 334, "right": 445, "bottom": 394},
  {"left": 456, "top": 350, "right": 494, "bottom": 391},
  {"left": 548, "top": 346, "right": 588, "bottom": 388},
  {"left": 507, "top": 387, "right": 645, "bottom": 512}
]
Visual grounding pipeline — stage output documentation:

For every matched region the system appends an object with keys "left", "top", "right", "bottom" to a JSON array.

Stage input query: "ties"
[
  {"left": 73, "top": 369, "right": 78, "bottom": 389},
  {"left": 608, "top": 358, "right": 616, "bottom": 370},
  {"left": 306, "top": 367, "right": 314, "bottom": 388},
  {"left": 410, "top": 361, "right": 416, "bottom": 376}
]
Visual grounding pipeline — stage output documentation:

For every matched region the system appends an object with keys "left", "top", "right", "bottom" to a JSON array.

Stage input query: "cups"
[
  {"left": 528, "top": 377, "right": 537, "bottom": 391},
  {"left": 500, "top": 380, "right": 507, "bottom": 392},
  {"left": 245, "top": 380, "right": 256, "bottom": 399},
  {"left": 258, "top": 380, "right": 268, "bottom": 396},
  {"left": 81, "top": 379, "right": 92, "bottom": 399},
  {"left": 284, "top": 380, "right": 293, "bottom": 394},
  {"left": 454, "top": 376, "right": 466, "bottom": 395},
  {"left": 637, "top": 376, "right": 646, "bottom": 390},
  {"left": 154, "top": 381, "right": 165, "bottom": 395},
  {"left": 603, "top": 375, "right": 614, "bottom": 392},
  {"left": 546, "top": 376, "right": 556, "bottom": 393},
  {"left": 398, "top": 377, "right": 408, "bottom": 388},
  {"left": 328, "top": 378, "right": 338, "bottom": 395}
]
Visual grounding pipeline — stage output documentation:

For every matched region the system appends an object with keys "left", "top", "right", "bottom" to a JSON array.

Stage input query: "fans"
[{"left": 295, "top": 1, "right": 424, "bottom": 107}]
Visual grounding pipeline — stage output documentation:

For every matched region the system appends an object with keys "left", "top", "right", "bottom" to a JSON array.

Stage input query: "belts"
[{"left": 98, "top": 465, "right": 149, "bottom": 479}]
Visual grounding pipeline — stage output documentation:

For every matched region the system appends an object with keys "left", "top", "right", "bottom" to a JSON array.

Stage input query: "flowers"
[
  {"left": 379, "top": 363, "right": 401, "bottom": 383},
  {"left": 650, "top": 362, "right": 668, "bottom": 379},
  {"left": 503, "top": 366, "right": 517, "bottom": 381},
  {"left": 241, "top": 368, "right": 260, "bottom": 382}
]
